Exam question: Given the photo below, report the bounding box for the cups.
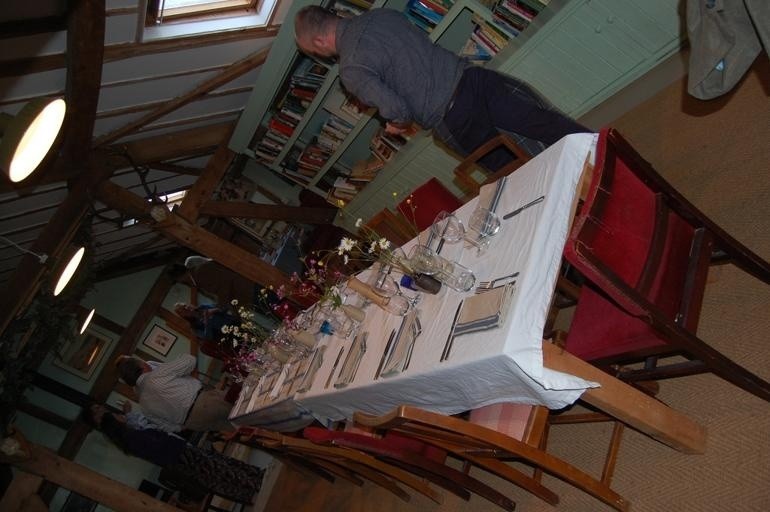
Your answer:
[439,261,480,294]
[467,207,503,236]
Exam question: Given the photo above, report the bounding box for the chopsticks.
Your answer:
[441,297,468,363]
[437,215,451,254]
[374,328,396,379]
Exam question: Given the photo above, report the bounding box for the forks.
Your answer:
[401,315,421,372]
[472,270,521,294]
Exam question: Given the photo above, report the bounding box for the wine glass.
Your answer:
[402,243,460,283]
[227,273,368,402]
[371,271,424,308]
[430,212,492,259]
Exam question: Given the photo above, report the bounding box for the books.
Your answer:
[252,0,551,205]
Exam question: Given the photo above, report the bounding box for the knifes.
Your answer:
[500,195,547,220]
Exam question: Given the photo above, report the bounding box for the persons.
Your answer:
[81,258,277,504]
[294,5,597,175]
